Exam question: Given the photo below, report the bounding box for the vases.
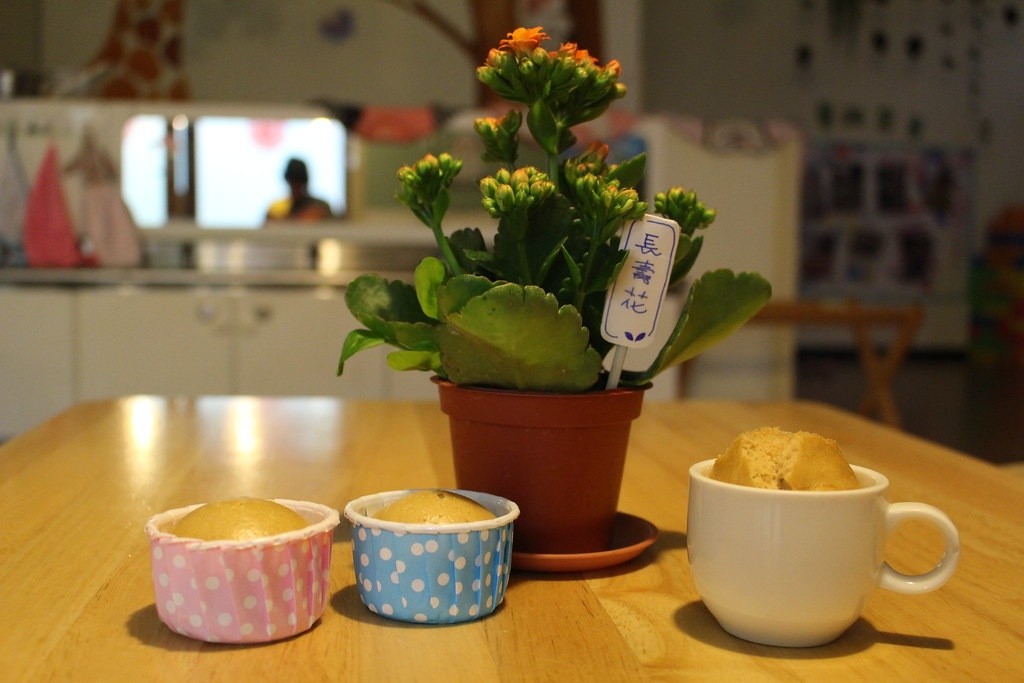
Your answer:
[428,372,662,572]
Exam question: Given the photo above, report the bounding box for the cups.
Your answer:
[684,457,958,649]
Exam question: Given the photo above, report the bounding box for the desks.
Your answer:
[1,390,1024,682]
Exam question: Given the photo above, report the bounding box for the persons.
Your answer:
[263,158,339,227]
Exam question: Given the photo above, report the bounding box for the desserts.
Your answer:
[146,494,340,644]
[343,487,519,623]
[705,426,863,491]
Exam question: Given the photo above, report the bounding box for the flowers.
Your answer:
[331,25,773,396]
